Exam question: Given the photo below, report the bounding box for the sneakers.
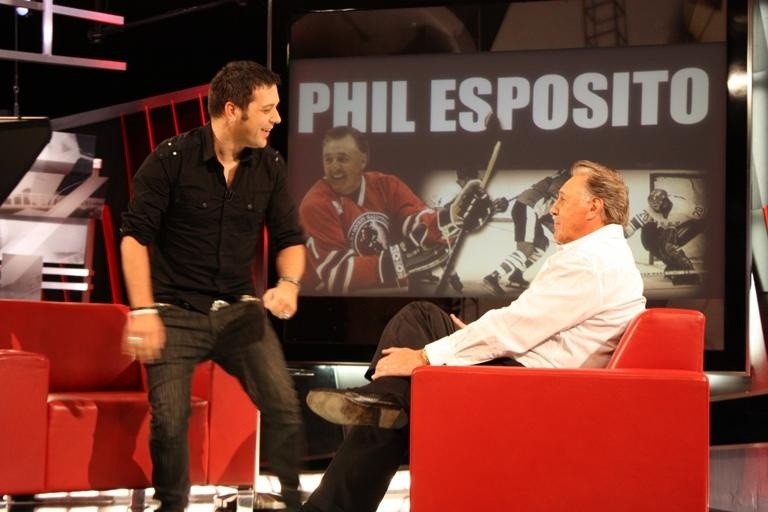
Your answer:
[483,274,505,295]
[509,272,529,287]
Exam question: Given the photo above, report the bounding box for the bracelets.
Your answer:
[420,346,431,365]
[127,304,164,313]
[279,277,301,288]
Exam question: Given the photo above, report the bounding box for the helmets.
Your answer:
[648,188,668,212]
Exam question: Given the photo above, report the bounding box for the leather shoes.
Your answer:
[305,389,409,431]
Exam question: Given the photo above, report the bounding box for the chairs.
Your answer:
[0,297,258,512]
[409,308,710,512]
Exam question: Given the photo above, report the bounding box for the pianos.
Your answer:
[0,127,114,302]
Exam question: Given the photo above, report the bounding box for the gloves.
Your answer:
[378,221,448,283]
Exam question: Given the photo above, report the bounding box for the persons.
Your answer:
[624,188,705,284]
[120,60,307,511]
[253,157,651,511]
[299,125,495,296]
[484,163,574,295]
